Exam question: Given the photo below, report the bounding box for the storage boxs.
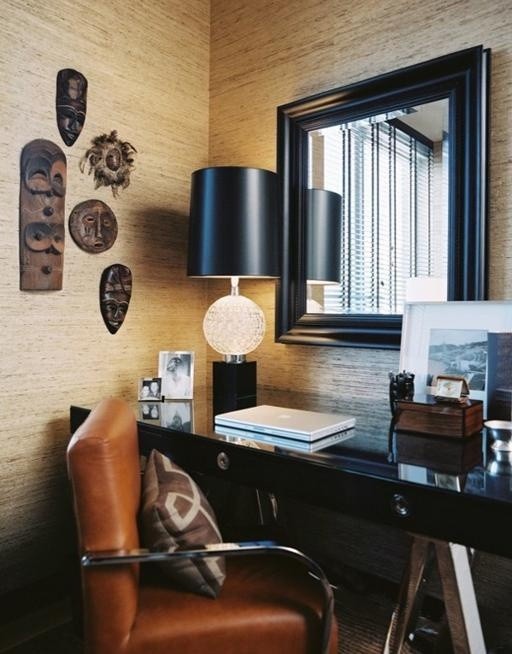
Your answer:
[396,392,484,439]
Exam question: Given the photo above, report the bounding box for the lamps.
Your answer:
[304,188,342,287]
[188,167,283,404]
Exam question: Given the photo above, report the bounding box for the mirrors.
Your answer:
[276,45,489,350]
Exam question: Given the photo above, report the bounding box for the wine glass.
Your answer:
[485,448,512,478]
[483,420,512,452]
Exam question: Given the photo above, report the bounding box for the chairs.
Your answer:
[66,399,338,653]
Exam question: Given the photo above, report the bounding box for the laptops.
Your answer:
[214,403,356,444]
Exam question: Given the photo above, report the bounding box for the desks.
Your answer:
[69,385,512,654]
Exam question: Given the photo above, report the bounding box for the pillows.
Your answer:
[140,449,226,600]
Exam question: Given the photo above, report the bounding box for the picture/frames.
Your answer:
[393,300,512,419]
[138,377,162,403]
[159,353,193,400]
